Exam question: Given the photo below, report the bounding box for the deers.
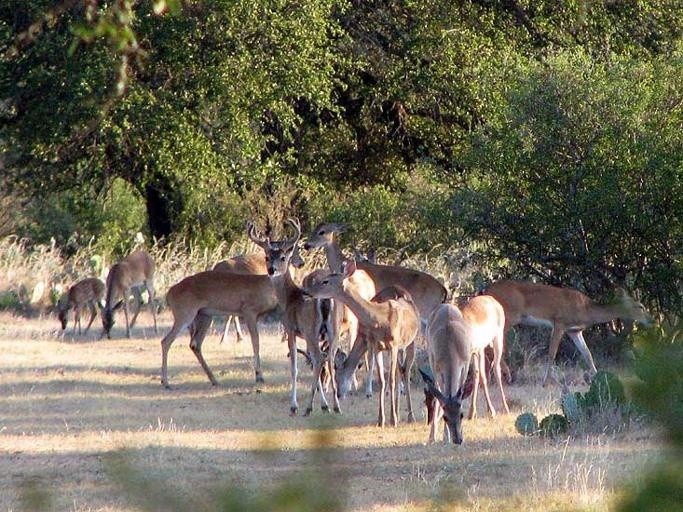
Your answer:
[96,249,159,342]
[159,214,656,447]
[56,277,108,336]
